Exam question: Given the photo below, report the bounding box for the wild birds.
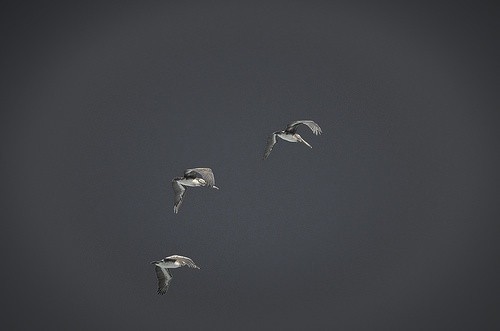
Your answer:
[265,120,323,158]
[172,167,219,214]
[150,254,201,296]
